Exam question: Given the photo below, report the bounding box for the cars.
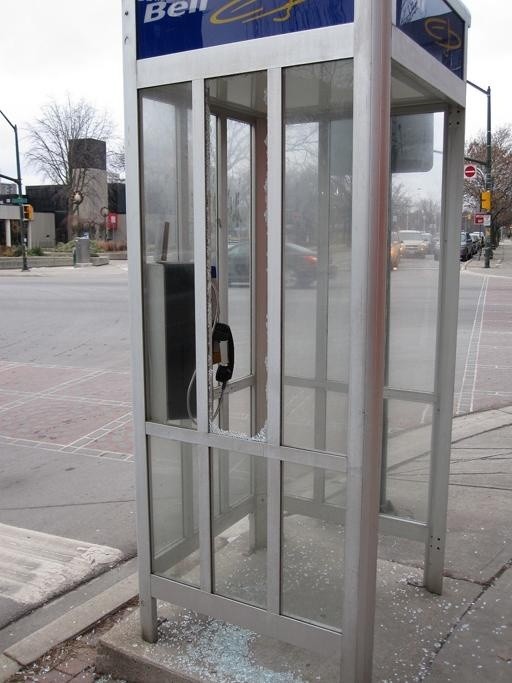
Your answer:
[210,239,335,289]
[390,228,486,272]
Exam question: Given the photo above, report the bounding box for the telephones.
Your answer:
[158,262,235,419]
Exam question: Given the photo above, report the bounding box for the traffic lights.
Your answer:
[480,189,491,212]
[23,205,33,220]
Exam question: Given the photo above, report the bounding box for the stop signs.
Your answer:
[463,164,477,179]
